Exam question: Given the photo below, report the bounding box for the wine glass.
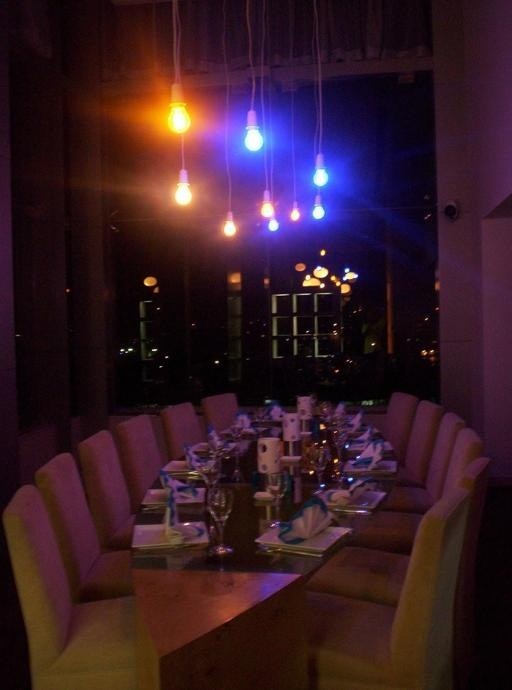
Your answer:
[207,485,235,557]
[268,471,291,530]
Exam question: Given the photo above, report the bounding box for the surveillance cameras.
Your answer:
[444,199,457,219]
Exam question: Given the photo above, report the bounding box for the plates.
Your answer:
[255,522,353,559]
[314,489,386,515]
[142,488,206,506]
[339,423,381,437]
[219,423,269,438]
[160,457,218,475]
[189,438,238,454]
[131,521,210,549]
[344,439,395,454]
[340,457,398,475]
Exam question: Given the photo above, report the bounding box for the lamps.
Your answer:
[166,0,329,238]
